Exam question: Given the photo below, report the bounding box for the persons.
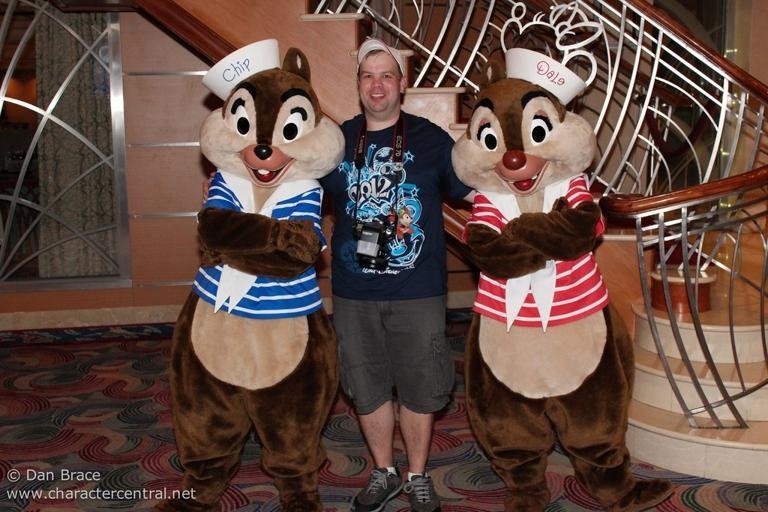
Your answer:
[198,38,480,512]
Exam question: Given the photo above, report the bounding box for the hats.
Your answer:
[358,38,405,75]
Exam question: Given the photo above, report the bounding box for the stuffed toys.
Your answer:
[446,44,677,511]
[167,35,341,512]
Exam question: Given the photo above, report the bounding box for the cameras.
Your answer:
[352,221,393,272]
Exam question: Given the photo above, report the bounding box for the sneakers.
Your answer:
[406,473,440,511]
[351,464,402,511]
